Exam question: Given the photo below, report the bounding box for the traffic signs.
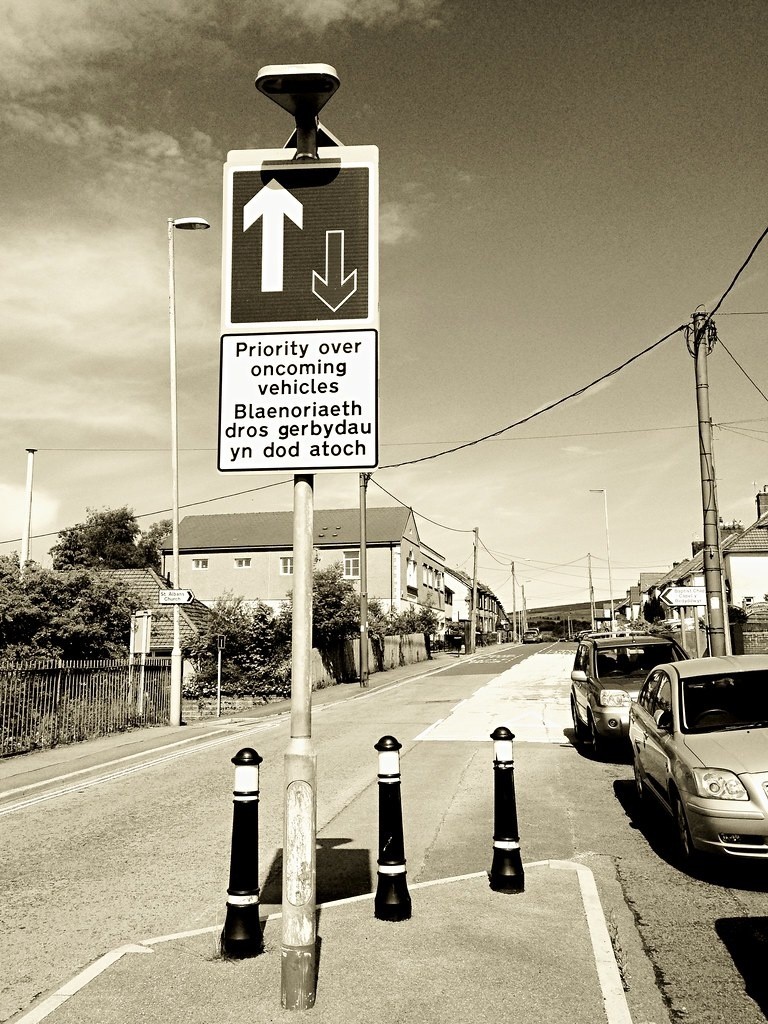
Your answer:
[659,586,708,607]
[158,589,194,604]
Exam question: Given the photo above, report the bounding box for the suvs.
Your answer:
[569,633,690,753]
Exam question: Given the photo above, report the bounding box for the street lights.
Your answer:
[251,61,343,1015]
[167,215,213,728]
[588,488,616,653]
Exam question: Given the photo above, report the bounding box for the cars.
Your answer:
[523,628,543,643]
[629,654,768,878]
[558,629,646,660]
